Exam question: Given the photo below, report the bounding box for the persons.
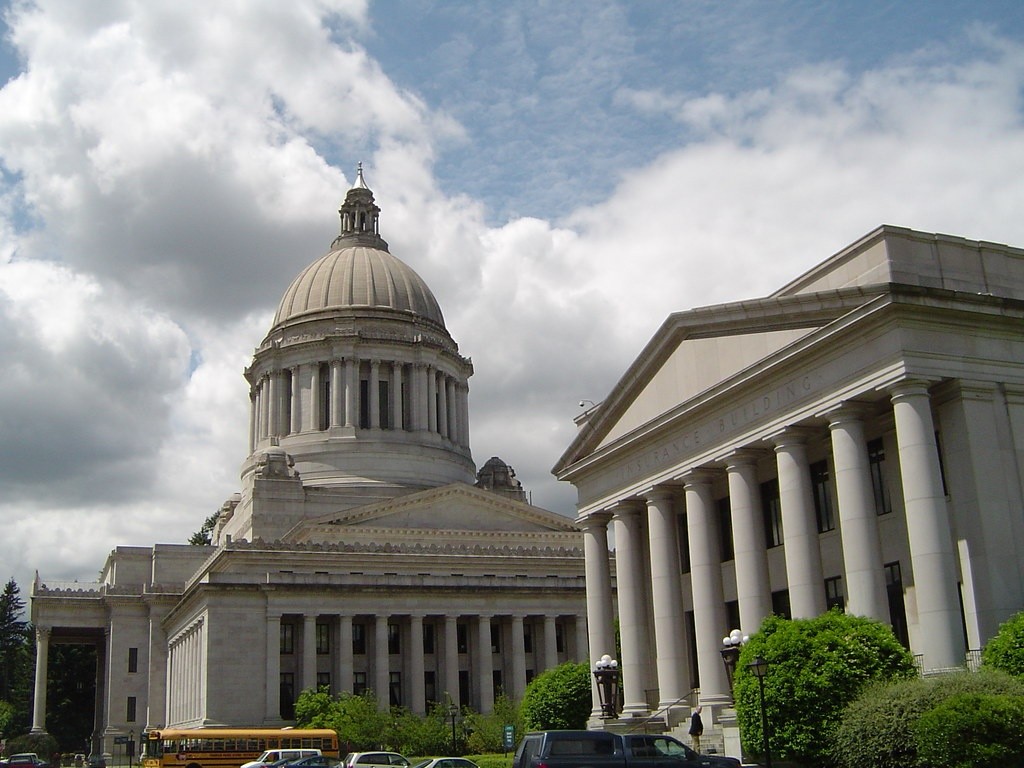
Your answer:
[688,705,703,753]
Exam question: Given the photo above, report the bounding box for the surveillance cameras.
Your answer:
[579,401,584,407]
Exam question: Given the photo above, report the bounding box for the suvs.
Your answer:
[338,751,414,768]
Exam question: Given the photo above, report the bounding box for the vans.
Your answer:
[240,748,322,768]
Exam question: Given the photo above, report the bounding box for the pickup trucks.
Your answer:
[511,728,743,768]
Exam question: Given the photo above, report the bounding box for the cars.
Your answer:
[406,757,481,768]
[0,753,49,768]
[260,755,342,768]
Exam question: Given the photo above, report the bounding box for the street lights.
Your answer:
[448,703,459,757]
[748,654,772,768]
[128,730,134,768]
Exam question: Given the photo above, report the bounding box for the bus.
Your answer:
[142,727,340,768]
